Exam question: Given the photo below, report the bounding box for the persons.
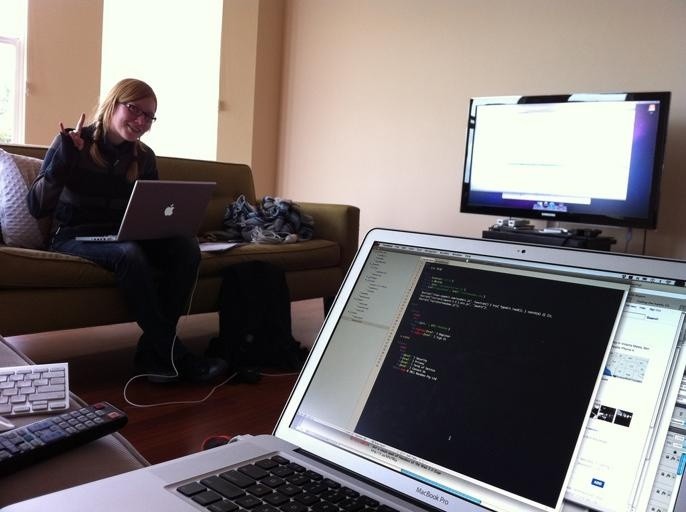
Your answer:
[25,77,218,390]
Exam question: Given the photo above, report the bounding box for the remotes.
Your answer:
[0,398,129,465]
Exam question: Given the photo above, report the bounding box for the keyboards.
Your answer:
[0,361,71,418]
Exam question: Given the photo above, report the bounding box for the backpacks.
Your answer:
[219,259,301,369]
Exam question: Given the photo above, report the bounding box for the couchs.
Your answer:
[0,143,360,367]
[0,333,153,512]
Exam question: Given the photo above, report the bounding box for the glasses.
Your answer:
[124,103,156,123]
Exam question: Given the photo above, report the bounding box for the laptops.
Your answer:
[0,229,686,512]
[73,178,219,243]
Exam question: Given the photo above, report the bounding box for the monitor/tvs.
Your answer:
[458,90,673,233]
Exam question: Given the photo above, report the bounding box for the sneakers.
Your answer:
[134,343,196,383]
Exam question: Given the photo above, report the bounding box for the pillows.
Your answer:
[0,148,50,252]
